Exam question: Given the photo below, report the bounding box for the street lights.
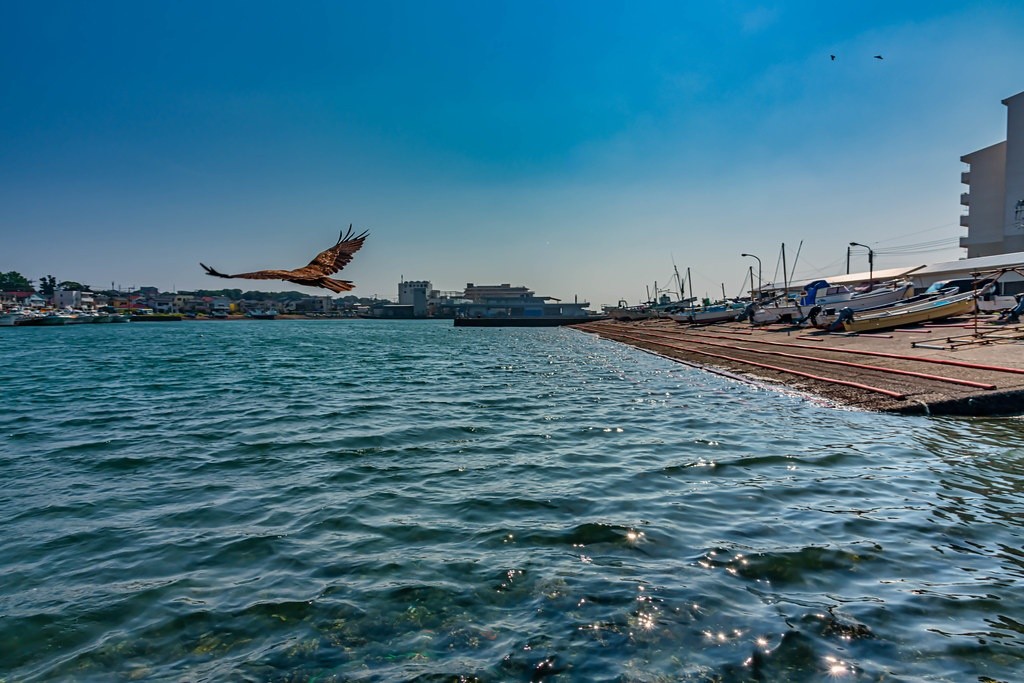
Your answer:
[742,254,761,303]
[850,242,873,284]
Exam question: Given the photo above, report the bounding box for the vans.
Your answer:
[924,278,999,315]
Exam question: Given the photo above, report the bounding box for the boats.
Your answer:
[975,295,1021,314]
[668,304,746,324]
[249,309,278,320]
[816,287,960,331]
[601,295,697,321]
[753,278,915,324]
[15,309,78,325]
[69,309,99,323]
[92,312,117,322]
[0,305,16,326]
[829,289,984,332]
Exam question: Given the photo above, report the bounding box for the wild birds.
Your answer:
[830,55,835,60]
[874,56,883,60]
